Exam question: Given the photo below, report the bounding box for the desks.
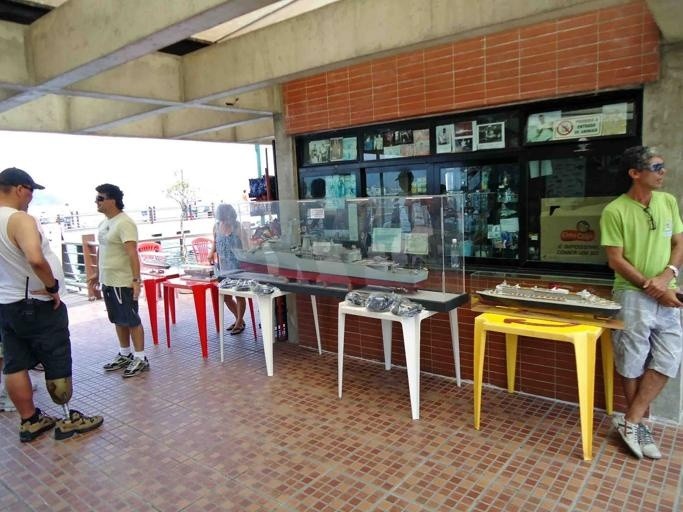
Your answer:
[336,300,461,420]
[472,306,615,461]
[218,284,322,377]
[163,277,219,358]
[143,273,179,348]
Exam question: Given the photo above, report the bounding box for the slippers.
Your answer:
[230,326,244,336]
[226,323,247,330]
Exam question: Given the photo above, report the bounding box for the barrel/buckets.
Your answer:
[444,170,461,192]
[460,238,475,257]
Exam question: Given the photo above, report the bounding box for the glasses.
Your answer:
[635,161,665,172]
[95,196,113,202]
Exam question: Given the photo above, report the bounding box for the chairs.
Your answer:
[137,242,162,298]
[191,237,215,266]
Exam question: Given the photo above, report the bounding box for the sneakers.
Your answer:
[638,423,663,459]
[121,355,151,378]
[32,383,38,391]
[103,352,134,371]
[53,409,106,441]
[611,412,643,458]
[0,390,17,412]
[15,410,62,441]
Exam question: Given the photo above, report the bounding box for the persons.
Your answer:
[207,204,249,335]
[1,167,104,442]
[85,184,149,378]
[390,171,414,228]
[536,114,554,133]
[599,146,683,461]
[311,178,338,230]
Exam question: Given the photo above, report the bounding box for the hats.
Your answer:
[0,167,46,192]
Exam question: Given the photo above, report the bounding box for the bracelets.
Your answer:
[664,264,679,277]
[132,278,141,283]
[45,279,59,293]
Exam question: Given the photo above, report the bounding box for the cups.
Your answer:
[302,235,311,250]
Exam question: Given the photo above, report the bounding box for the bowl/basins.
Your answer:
[309,244,335,256]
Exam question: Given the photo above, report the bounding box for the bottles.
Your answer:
[450,239,459,267]
